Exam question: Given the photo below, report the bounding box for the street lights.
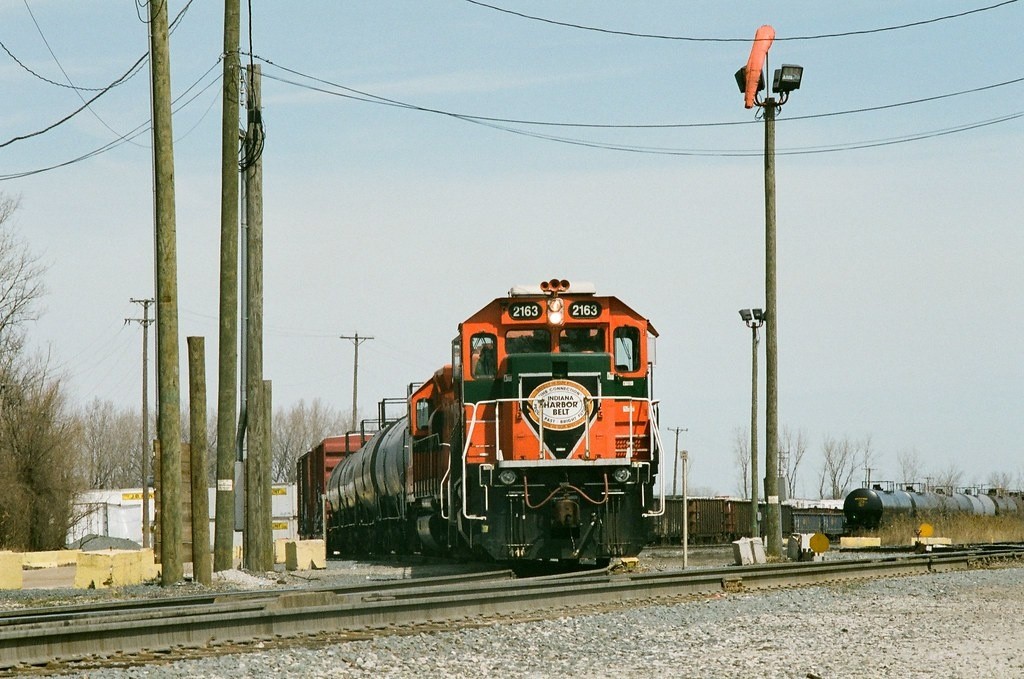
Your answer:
[733,62,805,560]
[737,308,766,536]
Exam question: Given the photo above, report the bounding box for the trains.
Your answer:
[296,275,671,580]
